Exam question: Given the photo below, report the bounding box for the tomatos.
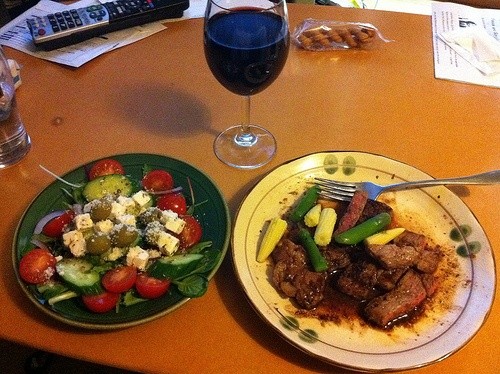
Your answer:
[155,192,186,215]
[142,169,174,196]
[101,266,138,292]
[82,291,121,312]
[41,211,75,236]
[89,158,125,180]
[19,249,57,284]
[135,271,172,298]
[168,215,202,249]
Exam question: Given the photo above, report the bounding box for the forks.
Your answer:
[313,169,500,203]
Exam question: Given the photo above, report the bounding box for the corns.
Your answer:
[304,203,322,227]
[364,227,405,246]
[314,208,337,246]
[256,218,288,262]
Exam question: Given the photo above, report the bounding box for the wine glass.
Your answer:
[203,0,291,170]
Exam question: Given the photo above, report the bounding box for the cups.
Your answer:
[0,48,31,170]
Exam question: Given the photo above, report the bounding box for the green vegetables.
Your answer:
[37,281,79,305]
[169,240,221,298]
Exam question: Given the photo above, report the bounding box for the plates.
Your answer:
[9,151,231,334]
[232,150,497,371]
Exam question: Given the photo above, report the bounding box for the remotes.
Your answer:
[27,0,190,52]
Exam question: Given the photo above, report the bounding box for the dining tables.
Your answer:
[0,3,500,374]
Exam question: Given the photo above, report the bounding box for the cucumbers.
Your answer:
[82,174,132,202]
[147,253,204,279]
[55,258,104,295]
